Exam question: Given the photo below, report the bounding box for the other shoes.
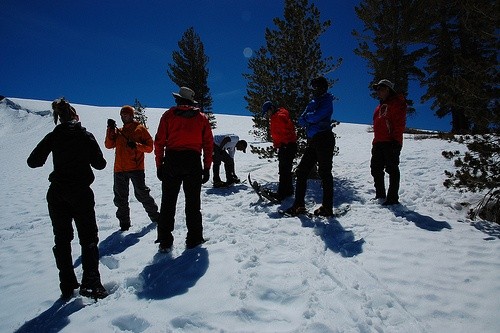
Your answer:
[185,239,205,249]
[59,268,80,299]
[158,241,173,252]
[80,271,109,299]
[227,176,240,184]
[314,206,333,216]
[286,205,306,214]
[213,181,228,187]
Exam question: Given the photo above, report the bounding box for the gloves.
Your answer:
[127,141,136,150]
[201,169,210,184]
[157,168,163,181]
[107,119,116,128]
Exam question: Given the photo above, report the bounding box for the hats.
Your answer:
[241,140,247,153]
[262,101,273,115]
[172,87,198,103]
[120,106,134,117]
[373,79,397,94]
[310,77,328,90]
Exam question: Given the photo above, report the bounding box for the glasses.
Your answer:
[375,86,384,91]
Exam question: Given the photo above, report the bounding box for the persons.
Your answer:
[154,87,214,249]
[212,134,247,188]
[278,75,335,218]
[262,101,297,201]
[27,97,108,301]
[370,79,407,206]
[105,105,160,231]
